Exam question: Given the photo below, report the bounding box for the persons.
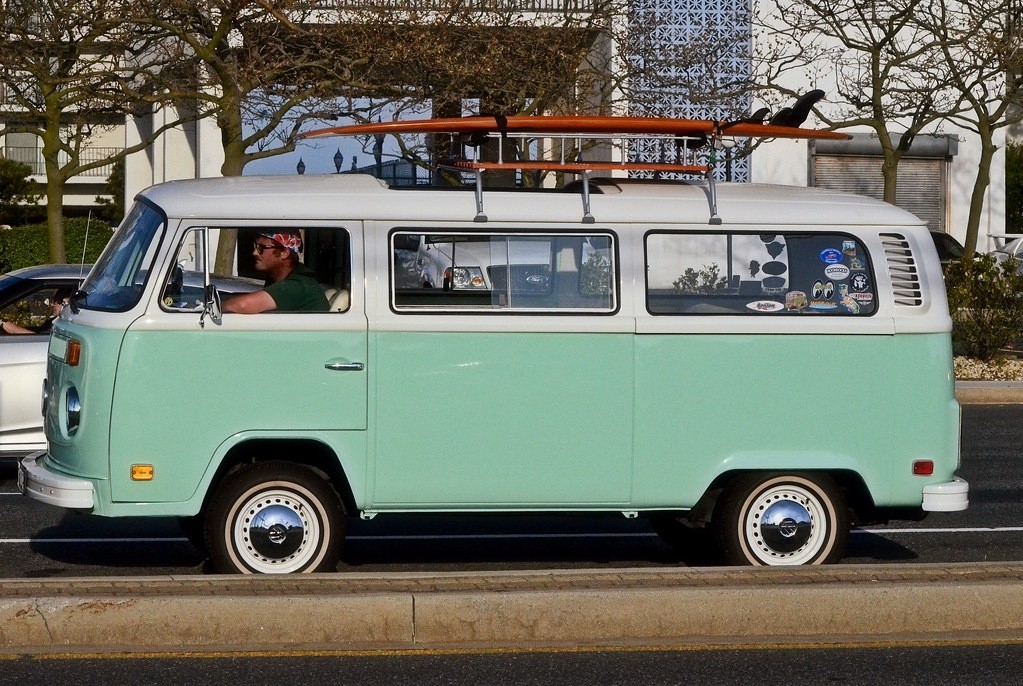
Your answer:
[0,287,76,334]
[221,227,330,314]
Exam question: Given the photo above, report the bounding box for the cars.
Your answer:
[930,229,979,282]
[985,237,1023,278]
[0,265,266,463]
[417,229,610,297]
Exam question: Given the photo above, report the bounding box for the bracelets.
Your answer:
[0,320,8,328]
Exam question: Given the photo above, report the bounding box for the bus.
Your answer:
[29,116,971,577]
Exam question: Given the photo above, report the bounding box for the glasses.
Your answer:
[253,241,284,255]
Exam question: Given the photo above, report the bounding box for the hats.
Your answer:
[259,229,303,257]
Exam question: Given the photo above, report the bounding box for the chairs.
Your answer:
[319,283,350,312]
